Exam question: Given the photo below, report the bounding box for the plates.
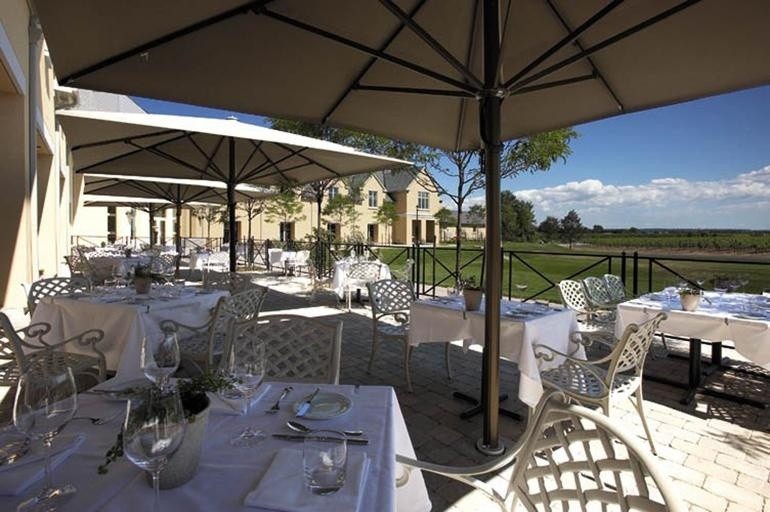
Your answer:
[100,377,153,403]
[292,390,354,421]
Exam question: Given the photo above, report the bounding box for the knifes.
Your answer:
[271,433,370,447]
[295,387,320,419]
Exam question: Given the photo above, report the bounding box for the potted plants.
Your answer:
[446,270,487,310]
[95,377,241,489]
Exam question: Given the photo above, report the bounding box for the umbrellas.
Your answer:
[25,0,770,446]
[81,172,278,273]
[82,194,220,249]
[53,112,416,292]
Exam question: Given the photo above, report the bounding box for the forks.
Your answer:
[68,408,120,425]
[263,386,293,417]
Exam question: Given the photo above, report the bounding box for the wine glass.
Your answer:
[121,388,186,512]
[111,262,126,291]
[10,361,80,512]
[139,331,182,395]
[229,338,269,449]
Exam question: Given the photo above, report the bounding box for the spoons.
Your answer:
[285,419,364,436]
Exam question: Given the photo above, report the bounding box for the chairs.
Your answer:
[393,387,673,510]
[29,275,92,320]
[62,243,418,375]
[555,273,668,368]
[0,309,109,421]
[364,279,453,393]
[213,314,344,383]
[532,308,668,472]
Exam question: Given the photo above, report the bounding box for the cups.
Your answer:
[300,427,348,497]
